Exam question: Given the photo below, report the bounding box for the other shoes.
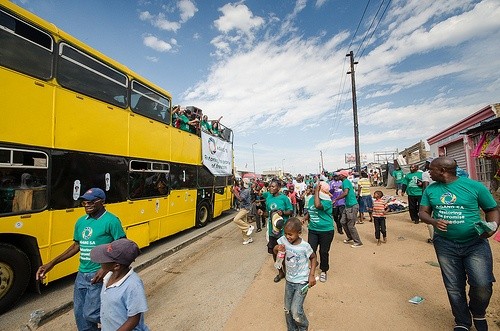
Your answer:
[257,228,261,232]
[351,243,363,248]
[344,239,354,243]
[338,228,343,234]
[454,324,470,331]
[320,272,326,282]
[468,304,488,331]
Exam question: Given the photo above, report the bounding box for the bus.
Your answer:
[0,0,234,315]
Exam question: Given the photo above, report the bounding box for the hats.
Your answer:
[242,178,250,189]
[335,170,349,177]
[317,181,330,200]
[90,238,139,264]
[79,189,106,202]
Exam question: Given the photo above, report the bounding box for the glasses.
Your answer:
[82,199,101,206]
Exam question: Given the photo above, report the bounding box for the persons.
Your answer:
[368,170,378,187]
[454,160,469,178]
[230,174,270,245]
[36,189,127,331]
[283,172,318,218]
[348,172,373,224]
[419,156,500,331]
[392,166,404,195]
[421,160,433,243]
[369,191,390,244]
[12,173,34,212]
[322,169,344,234]
[401,165,424,224]
[258,180,293,284]
[273,218,317,331]
[91,239,150,331]
[335,171,363,248]
[137,94,224,140]
[301,181,334,282]
[150,173,180,194]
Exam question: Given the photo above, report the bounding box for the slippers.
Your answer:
[274,275,284,282]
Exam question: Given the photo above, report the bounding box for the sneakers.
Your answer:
[247,225,254,235]
[243,237,253,245]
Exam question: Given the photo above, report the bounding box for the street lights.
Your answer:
[282,158,286,172]
[252,142,258,174]
